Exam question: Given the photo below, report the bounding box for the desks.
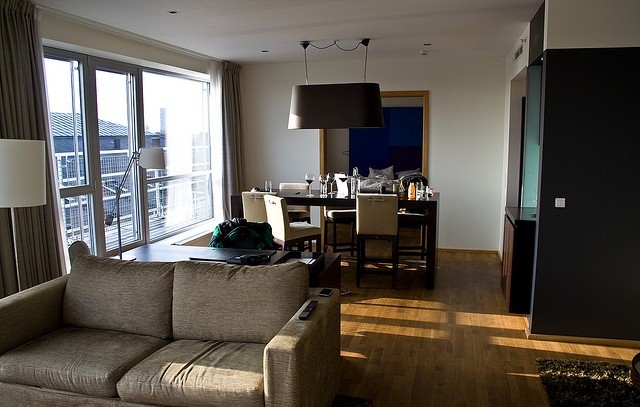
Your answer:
[110,243,342,294]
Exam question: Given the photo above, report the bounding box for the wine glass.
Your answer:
[319,174,327,196]
[327,172,336,195]
[338,172,348,196]
[304,173,316,197]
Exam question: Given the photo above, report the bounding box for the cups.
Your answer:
[264,180,273,192]
[423,186,427,199]
[427,187,434,197]
[416,181,423,201]
[380,182,387,194]
[393,184,400,193]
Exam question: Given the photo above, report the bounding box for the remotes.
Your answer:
[298,298,319,319]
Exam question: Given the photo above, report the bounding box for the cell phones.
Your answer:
[317,285,334,297]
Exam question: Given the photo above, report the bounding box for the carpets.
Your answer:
[534,356,639,406]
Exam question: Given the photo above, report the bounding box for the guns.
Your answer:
[189,254,270,266]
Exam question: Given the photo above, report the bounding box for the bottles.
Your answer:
[349,167,361,197]
[407,182,416,200]
[398,179,405,193]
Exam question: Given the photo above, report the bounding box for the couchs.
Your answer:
[0,253,343,407]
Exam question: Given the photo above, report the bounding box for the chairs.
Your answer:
[241,191,276,223]
[278,182,313,252]
[319,181,356,256]
[354,193,400,290]
[263,193,322,253]
[399,218,425,262]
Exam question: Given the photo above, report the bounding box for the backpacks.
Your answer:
[207,217,274,250]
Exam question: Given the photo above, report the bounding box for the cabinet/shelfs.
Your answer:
[500,213,521,315]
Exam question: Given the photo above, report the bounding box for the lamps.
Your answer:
[1,139,47,292]
[106,147,166,261]
[287,39,386,130]
